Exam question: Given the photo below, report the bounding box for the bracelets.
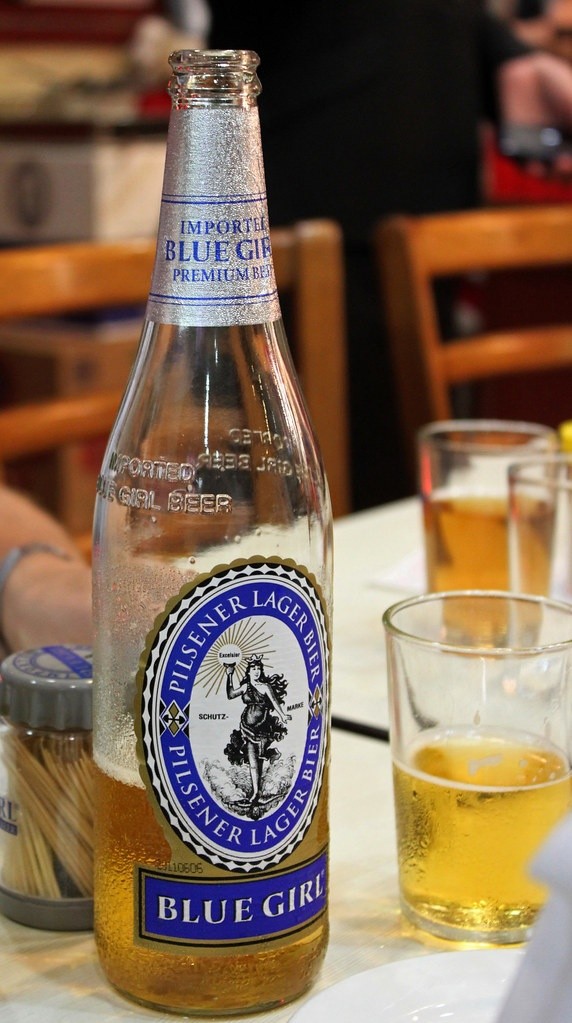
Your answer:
[0,542,68,591]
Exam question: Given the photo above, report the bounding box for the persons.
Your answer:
[0,475,97,654]
[189,0,572,516]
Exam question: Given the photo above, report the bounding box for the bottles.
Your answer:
[90,46,339,1020]
[0,646,93,931]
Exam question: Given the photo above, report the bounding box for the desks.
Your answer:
[0,422,572,1023]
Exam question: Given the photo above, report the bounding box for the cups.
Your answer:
[419,418,559,658]
[507,453,571,700]
[382,590,572,946]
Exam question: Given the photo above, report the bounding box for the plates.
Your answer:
[285,949,527,1023]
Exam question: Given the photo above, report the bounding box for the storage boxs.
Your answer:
[0,114,171,328]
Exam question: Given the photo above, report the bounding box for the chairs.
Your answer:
[0,221,347,576]
[378,207,572,492]
